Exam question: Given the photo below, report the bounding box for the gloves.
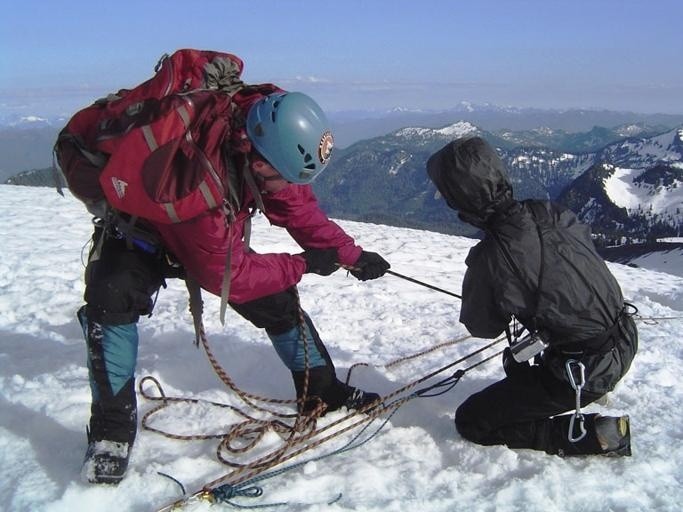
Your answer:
[300,247,339,277]
[350,249,390,282]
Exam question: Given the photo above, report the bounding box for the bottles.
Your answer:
[508,331,549,364]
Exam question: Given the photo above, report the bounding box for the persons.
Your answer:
[421,134,638,459]
[74,76,393,489]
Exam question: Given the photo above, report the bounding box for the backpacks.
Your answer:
[51,47,242,218]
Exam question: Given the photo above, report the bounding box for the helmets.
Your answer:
[246,91,333,186]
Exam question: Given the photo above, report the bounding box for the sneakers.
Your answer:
[83,417,136,484]
[293,371,380,415]
[594,415,631,456]
